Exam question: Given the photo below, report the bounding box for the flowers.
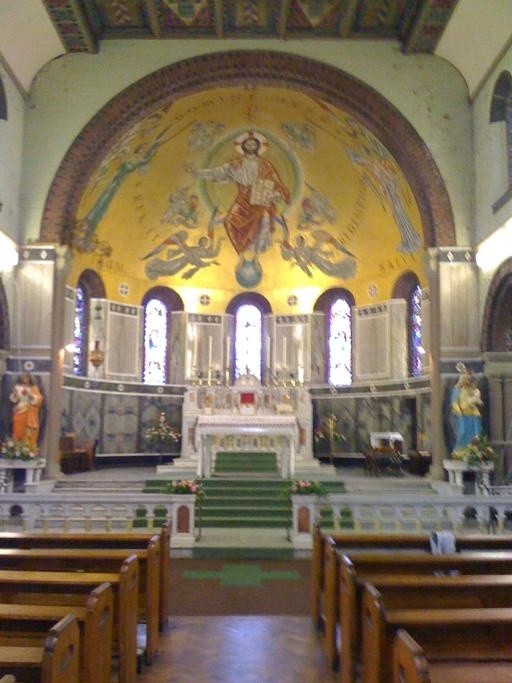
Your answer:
[1,439,38,459]
[168,480,200,493]
[454,434,492,463]
[289,479,319,494]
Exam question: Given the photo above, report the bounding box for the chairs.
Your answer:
[60,433,81,472]
[82,438,98,470]
[363,446,430,476]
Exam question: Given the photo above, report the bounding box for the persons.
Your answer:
[9,371,43,457]
[452,373,484,460]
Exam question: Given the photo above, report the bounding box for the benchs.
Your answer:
[308,524,512,683]
[0,529,171,683]
